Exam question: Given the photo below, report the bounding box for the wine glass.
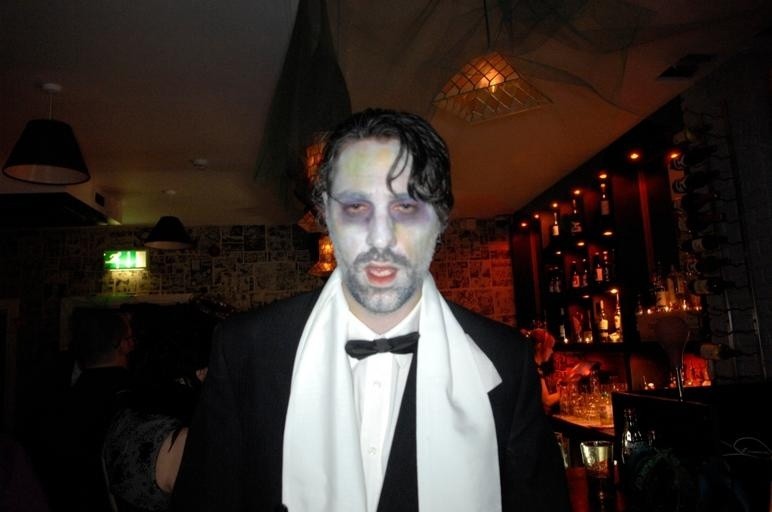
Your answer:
[559,361,628,425]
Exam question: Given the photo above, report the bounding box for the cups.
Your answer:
[579,440,614,490]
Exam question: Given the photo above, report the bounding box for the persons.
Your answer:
[25,309,207,511]
[525,327,563,415]
[170,105,572,511]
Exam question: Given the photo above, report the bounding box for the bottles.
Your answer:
[558,308,567,345]
[569,256,588,289]
[594,249,609,284]
[580,294,595,344]
[548,264,563,294]
[596,299,608,341]
[621,406,656,464]
[598,183,610,222]
[550,211,560,245]
[569,197,582,237]
[613,293,624,336]
[648,259,688,310]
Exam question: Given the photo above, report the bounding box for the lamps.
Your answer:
[141,189,197,250]
[3,83,91,186]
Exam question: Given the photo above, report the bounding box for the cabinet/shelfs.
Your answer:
[523,173,639,352]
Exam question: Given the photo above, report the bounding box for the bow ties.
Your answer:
[344,331,421,360]
